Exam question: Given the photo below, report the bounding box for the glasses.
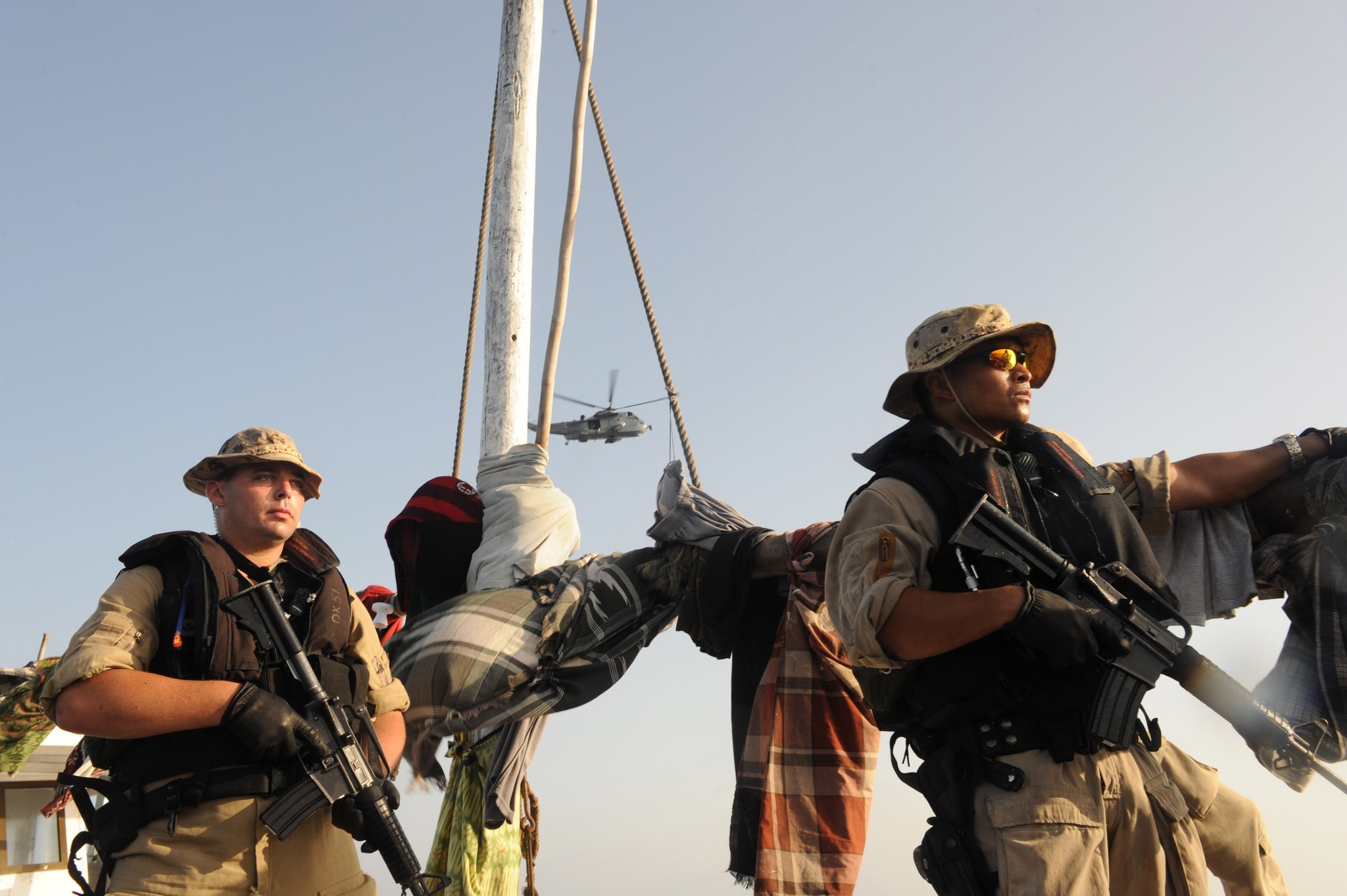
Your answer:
[942,347,1027,371]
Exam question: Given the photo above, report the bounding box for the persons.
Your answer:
[39,427,412,896]
[823,303,1347,895]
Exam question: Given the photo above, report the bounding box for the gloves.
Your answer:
[216,680,330,764]
[331,778,402,854]
[1299,427,1347,461]
[1001,580,1132,672]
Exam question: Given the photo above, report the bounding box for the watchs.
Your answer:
[1273,433,1306,474]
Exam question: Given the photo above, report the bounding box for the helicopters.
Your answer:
[528,367,669,446]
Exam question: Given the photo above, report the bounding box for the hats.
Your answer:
[881,302,1056,419]
[182,426,322,502]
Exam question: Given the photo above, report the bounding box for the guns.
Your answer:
[941,492,1347,805]
[219,582,454,896]
